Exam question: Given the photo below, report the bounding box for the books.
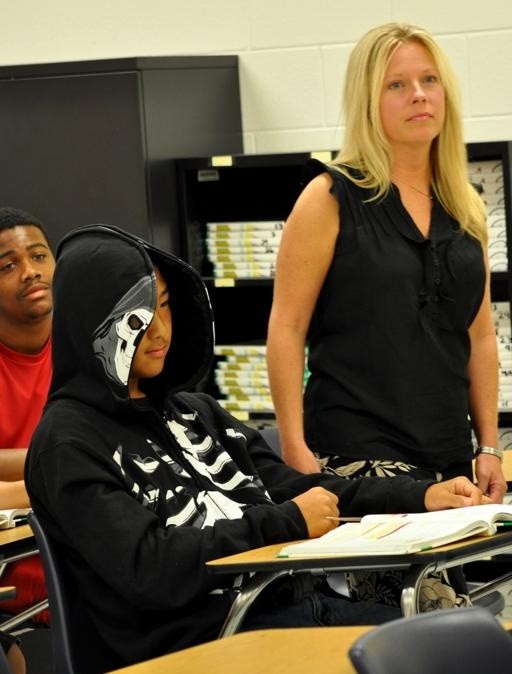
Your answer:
[276,502,512,561]
[205,220,284,280]
[493,301,512,412]
[213,345,313,413]
[0,507,32,529]
[469,161,509,272]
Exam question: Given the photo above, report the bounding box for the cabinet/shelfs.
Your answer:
[175,141,511,451]
[1,55,244,258]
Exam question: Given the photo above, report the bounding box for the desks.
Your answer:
[1,505,511,673]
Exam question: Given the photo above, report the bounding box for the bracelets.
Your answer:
[476,446,504,462]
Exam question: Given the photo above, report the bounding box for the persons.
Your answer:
[265,20,507,614]
[1,632,27,673]
[24,223,494,673]
[0,208,56,624]
